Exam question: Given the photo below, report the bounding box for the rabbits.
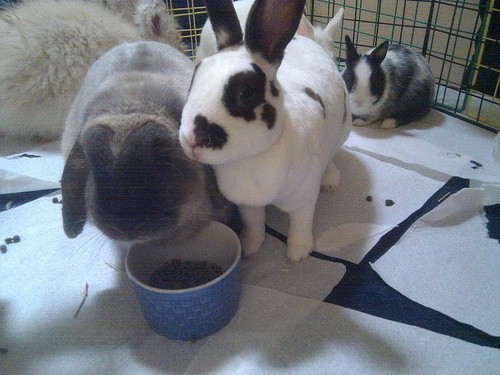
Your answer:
[341,34,436,129]
[0,0,352,265]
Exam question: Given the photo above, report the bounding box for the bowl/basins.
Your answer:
[126,220,242,341]
